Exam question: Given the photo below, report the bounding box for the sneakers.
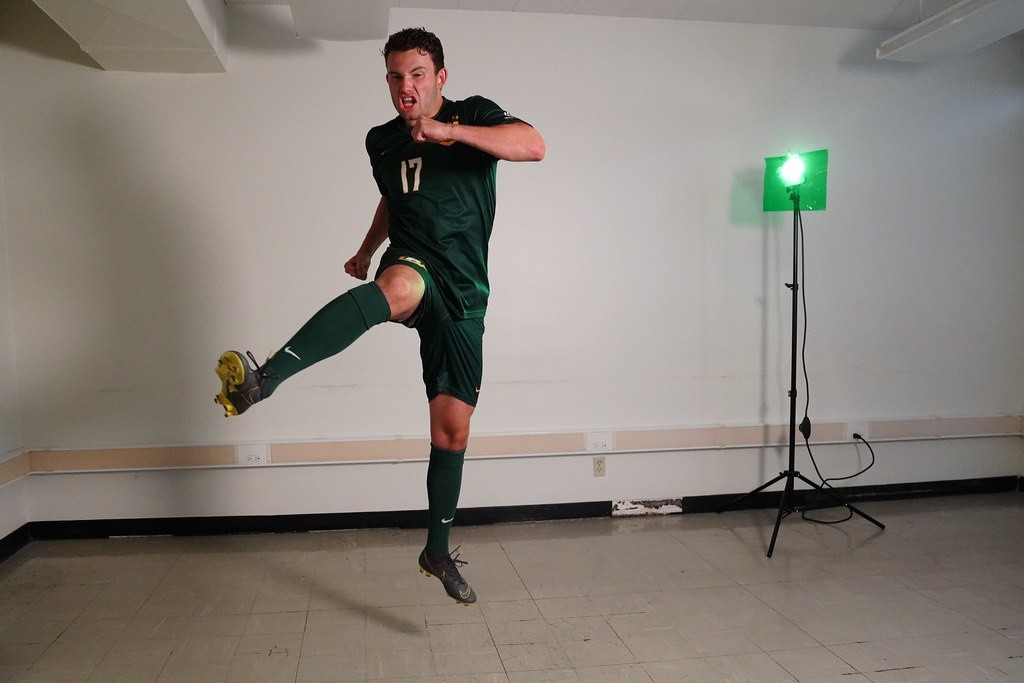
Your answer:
[215,350,277,417]
[418,542,477,607]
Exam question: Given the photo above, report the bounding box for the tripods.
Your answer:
[718,195,886,557]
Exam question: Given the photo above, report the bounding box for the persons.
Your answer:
[215,27,546,607]
